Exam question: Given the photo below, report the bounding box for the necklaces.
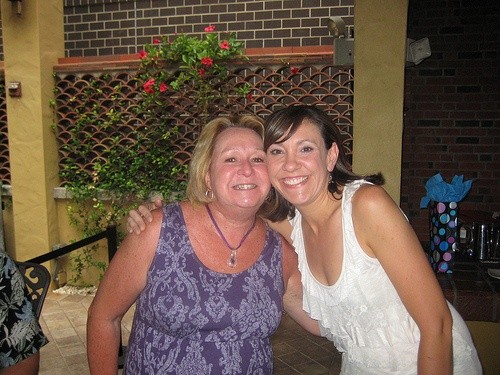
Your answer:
[206,204,257,266]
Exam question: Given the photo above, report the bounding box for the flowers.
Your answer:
[137,25,243,94]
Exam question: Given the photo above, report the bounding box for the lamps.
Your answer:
[326,15,354,67]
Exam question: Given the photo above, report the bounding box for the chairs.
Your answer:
[13,259,53,319]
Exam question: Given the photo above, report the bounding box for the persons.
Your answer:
[126,105,483,375]
[87,115,321,375]
[0,249,50,375]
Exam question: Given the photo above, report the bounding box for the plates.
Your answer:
[488,268,500,280]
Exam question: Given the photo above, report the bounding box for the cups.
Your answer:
[459,218,500,262]
